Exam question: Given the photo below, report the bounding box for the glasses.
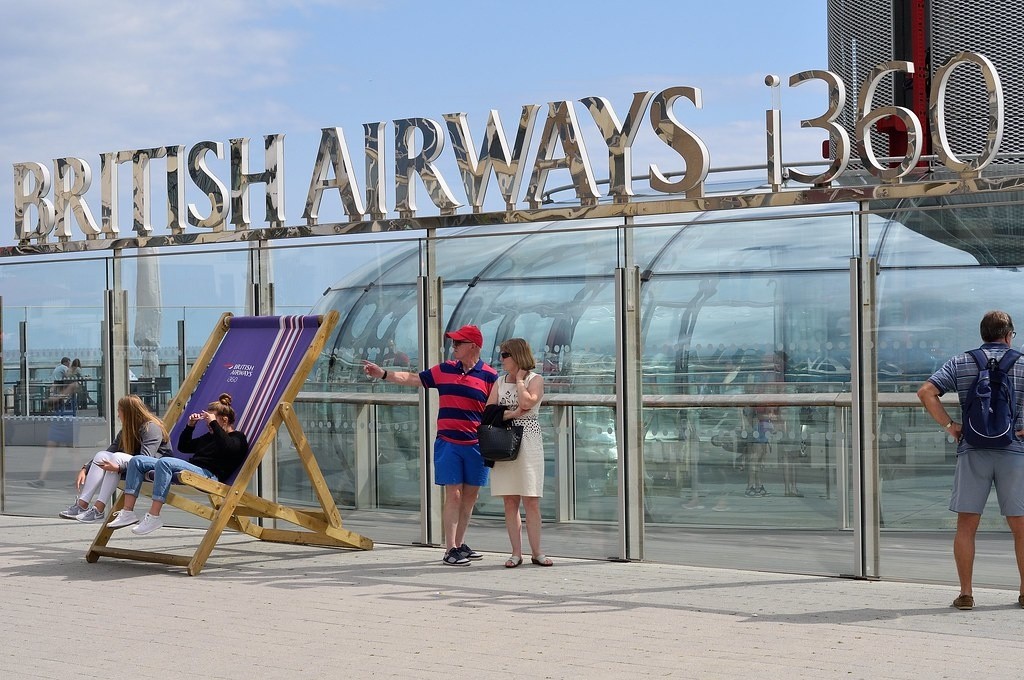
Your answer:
[452,340,473,346]
[1012,331,1016,338]
[499,352,511,359]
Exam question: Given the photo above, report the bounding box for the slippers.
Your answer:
[530,554,553,566]
[505,555,523,568]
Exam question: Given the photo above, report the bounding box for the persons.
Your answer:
[382,339,409,393]
[49,356,95,410]
[652,350,804,509]
[59,394,174,524]
[917,311,1024,610]
[486,338,553,567]
[362,326,498,566]
[107,392,248,535]
[25,379,80,488]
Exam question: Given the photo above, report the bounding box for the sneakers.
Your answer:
[106,509,140,529]
[682,500,705,510]
[26,478,45,488]
[76,505,105,523]
[712,502,728,512]
[132,513,162,535]
[953,594,973,610]
[59,500,90,519]
[1018,595,1024,609]
[744,483,772,498]
[63,480,75,488]
[443,543,484,566]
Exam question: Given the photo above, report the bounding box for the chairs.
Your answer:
[129,375,173,415]
[85,310,374,576]
[15,379,88,415]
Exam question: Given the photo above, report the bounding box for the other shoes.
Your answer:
[89,399,95,404]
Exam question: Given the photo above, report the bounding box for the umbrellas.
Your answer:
[135,247,161,378]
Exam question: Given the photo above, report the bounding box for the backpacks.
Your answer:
[962,349,1024,450]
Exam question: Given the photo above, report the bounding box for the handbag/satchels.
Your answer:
[478,410,524,461]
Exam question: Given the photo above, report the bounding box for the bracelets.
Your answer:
[517,379,524,383]
[118,464,120,475]
[381,370,388,379]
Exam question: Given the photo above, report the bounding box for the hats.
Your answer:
[445,325,483,349]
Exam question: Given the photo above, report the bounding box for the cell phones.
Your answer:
[92,462,108,466]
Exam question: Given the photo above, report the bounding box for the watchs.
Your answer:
[943,420,954,429]
